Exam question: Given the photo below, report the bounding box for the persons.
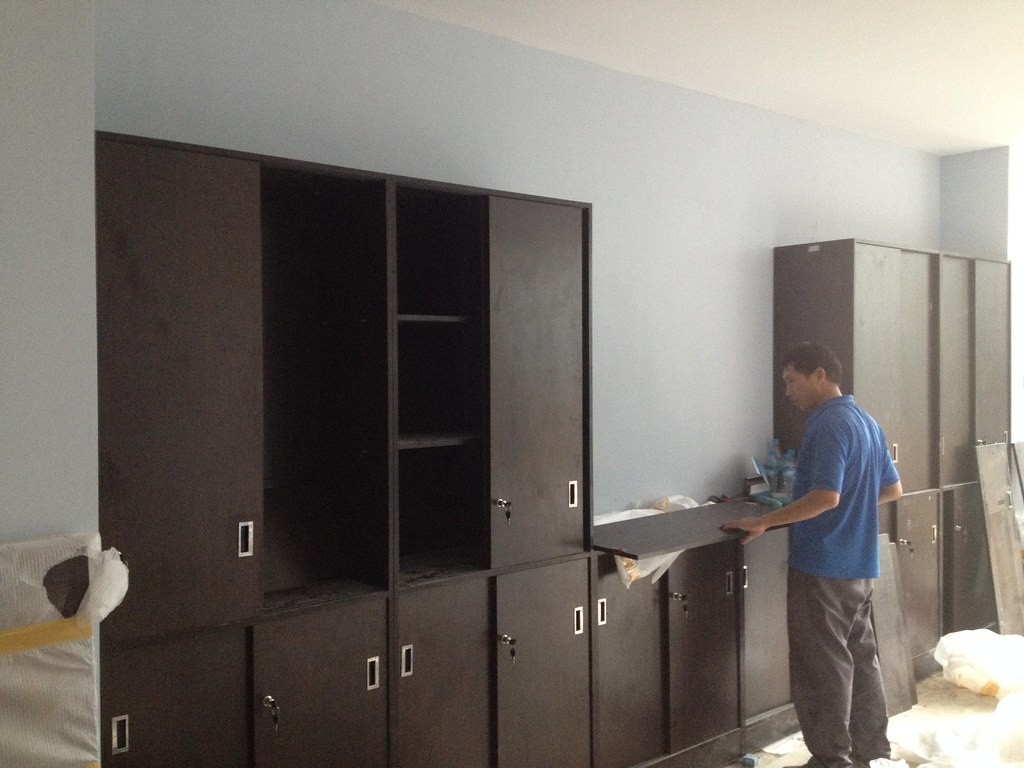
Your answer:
[718,341,904,768]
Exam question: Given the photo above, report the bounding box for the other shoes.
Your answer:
[782,752,871,768]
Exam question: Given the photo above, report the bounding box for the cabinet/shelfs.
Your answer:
[773,239,1011,494]
[391,174,594,582]
[896,492,941,658]
[944,488,994,635]
[664,526,791,755]
[101,553,665,768]
[96,130,393,643]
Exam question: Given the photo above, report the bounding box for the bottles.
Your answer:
[766,439,798,496]
